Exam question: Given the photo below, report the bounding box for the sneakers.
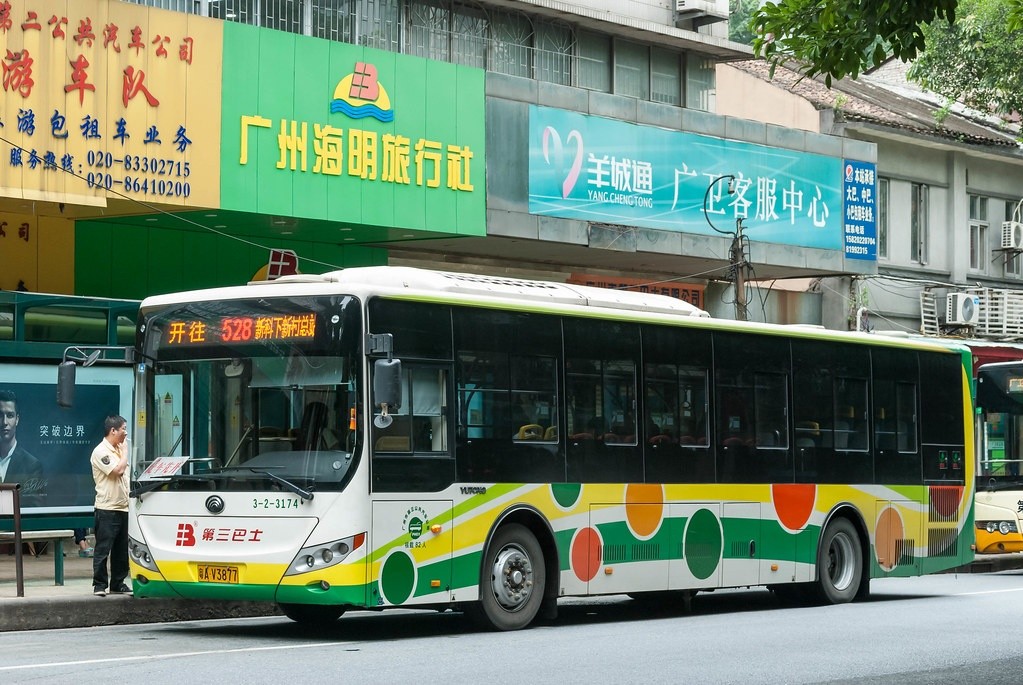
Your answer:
[94,584,134,596]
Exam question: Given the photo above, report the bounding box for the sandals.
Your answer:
[78,547,95,557]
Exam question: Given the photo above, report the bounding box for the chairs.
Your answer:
[372,413,432,453]
[490,393,912,455]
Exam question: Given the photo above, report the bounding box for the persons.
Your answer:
[74,529,95,557]
[292,402,330,450]
[0,390,44,507]
[88,415,134,596]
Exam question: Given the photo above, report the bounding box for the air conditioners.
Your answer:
[1000,221,1022,251]
[675,0,731,20]
[945,292,980,326]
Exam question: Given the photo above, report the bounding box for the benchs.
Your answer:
[0,529,75,586]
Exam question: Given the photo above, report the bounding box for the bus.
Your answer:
[51,260,976,634]
[968,355,1023,571]
